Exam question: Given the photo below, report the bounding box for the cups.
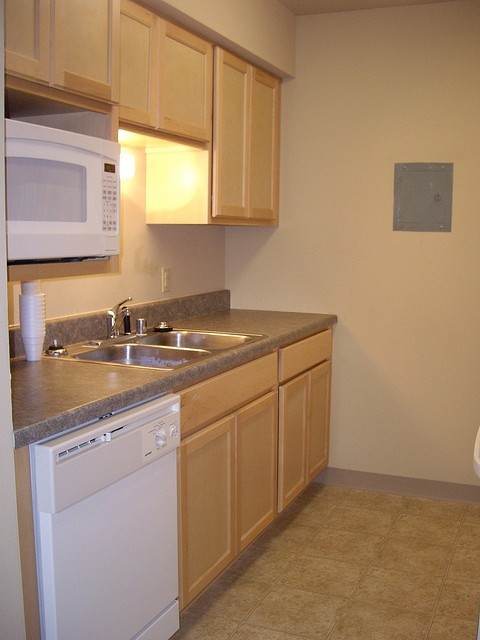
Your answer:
[19,280,45,331]
[19,331,46,362]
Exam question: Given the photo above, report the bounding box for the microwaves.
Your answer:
[5,119,122,260]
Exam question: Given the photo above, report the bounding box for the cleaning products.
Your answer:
[124,308,131,335]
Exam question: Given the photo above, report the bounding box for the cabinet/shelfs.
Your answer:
[179,353,280,605]
[2,0,117,117]
[145,46,280,227]
[118,1,216,148]
[279,328,333,512]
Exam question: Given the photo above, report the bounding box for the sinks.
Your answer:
[70,344,210,370]
[110,332,252,350]
[106,297,133,339]
